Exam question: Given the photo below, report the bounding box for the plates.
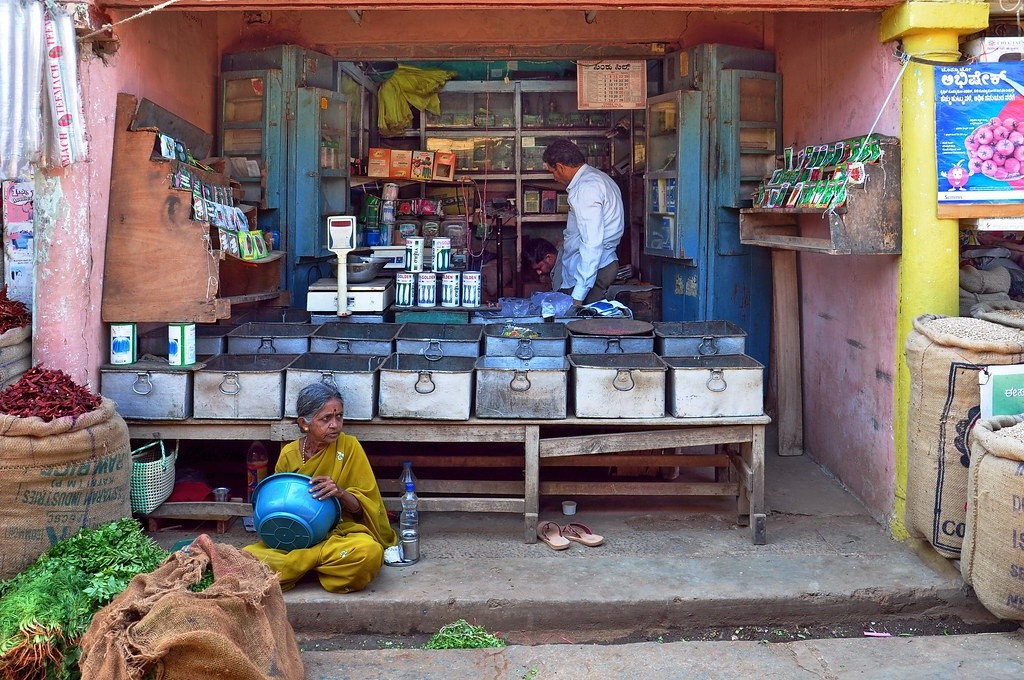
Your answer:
[384,558,419,567]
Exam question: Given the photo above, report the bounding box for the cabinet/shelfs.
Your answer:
[101,92,284,323]
[378,79,632,310]
[220,48,376,310]
[719,68,782,213]
[643,90,704,262]
[740,133,903,255]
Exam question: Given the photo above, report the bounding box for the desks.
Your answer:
[120,414,771,546]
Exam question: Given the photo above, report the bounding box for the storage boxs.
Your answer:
[367,148,455,182]
[426,187,474,216]
[100,312,764,422]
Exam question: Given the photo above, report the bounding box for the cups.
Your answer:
[562,501,577,515]
[212,487,232,503]
[398,528,419,562]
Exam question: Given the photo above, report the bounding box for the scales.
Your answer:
[306,215,396,317]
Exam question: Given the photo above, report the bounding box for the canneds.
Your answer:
[354,182,399,246]
[395,236,482,309]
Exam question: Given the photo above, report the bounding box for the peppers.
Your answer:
[0,362,103,421]
[0,284,32,335]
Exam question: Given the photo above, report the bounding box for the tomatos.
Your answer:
[964,117,1024,180]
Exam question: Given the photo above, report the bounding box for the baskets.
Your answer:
[130,439,180,515]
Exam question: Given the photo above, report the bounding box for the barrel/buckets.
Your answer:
[109,322,139,366]
[168,322,196,367]
[354,182,399,247]
[395,236,482,307]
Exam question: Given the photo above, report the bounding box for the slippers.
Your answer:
[560,523,603,547]
[536,521,570,550]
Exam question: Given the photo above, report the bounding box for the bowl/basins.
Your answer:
[251,472,341,552]
[327,256,391,284]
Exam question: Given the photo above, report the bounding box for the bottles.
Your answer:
[321,140,329,169]
[399,482,419,532]
[329,141,339,169]
[422,215,441,248]
[440,214,467,248]
[399,460,417,517]
[523,92,557,114]
[350,157,367,176]
[394,214,421,246]
[245,440,268,504]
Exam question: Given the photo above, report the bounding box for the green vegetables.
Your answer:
[0,516,213,680]
[421,617,509,649]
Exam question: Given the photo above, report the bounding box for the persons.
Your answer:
[522,237,564,291]
[241,380,397,594]
[542,136,625,305]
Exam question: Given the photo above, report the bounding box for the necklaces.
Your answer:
[302,436,308,464]
